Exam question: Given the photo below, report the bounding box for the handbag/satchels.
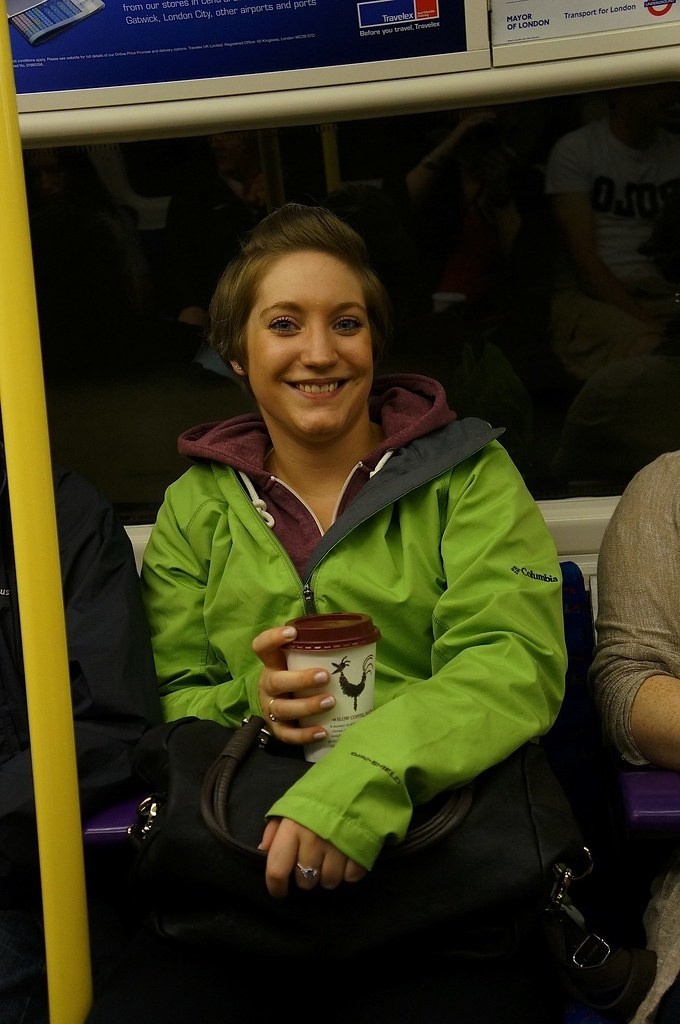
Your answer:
[132,717,657,1018]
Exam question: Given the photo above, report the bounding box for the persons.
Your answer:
[0,435,165,832]
[139,202,570,901]
[587,447,680,1024]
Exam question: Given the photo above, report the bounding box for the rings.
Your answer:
[268,696,281,722]
[296,861,322,881]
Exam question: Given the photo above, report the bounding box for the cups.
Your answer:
[283,616,379,762]
